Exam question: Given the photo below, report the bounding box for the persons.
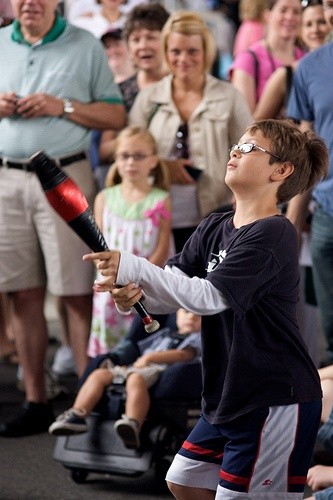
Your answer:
[82,119,324,500]
[0,0,333,500]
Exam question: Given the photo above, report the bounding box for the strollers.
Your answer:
[52,305,202,483]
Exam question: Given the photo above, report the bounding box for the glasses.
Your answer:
[230,142,284,161]
[173,121,189,160]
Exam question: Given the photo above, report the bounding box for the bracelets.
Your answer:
[57,94,75,119]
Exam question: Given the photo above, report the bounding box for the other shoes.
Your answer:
[114,414,139,449]
[4,402,52,438]
[49,409,89,435]
[1,349,20,368]
[17,372,69,402]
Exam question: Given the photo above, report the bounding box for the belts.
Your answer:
[0,151,86,172]
